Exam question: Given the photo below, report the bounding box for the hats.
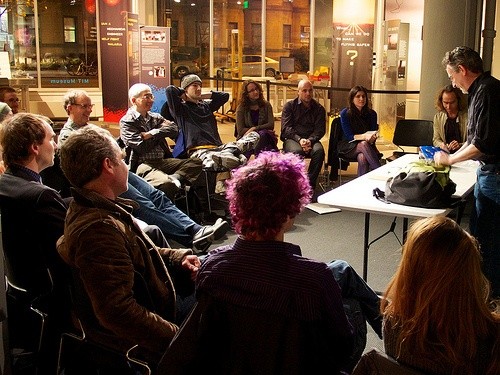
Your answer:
[181,74,202,89]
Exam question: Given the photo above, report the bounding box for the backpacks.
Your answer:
[373,159,462,209]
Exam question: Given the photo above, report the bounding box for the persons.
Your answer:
[192,150,383,375]
[380,216,500,375]
[281,79,327,202]
[0,74,278,375]
[434,46,500,308]
[433,84,468,153]
[336,85,381,178]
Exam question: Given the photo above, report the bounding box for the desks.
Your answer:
[0,75,35,113]
[316,154,484,281]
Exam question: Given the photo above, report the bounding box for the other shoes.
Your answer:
[148,177,182,194]
[212,152,240,169]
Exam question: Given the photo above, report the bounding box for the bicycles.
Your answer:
[65,56,98,79]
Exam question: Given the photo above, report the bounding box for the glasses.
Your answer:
[71,103,95,109]
[140,95,155,99]
[3,99,21,104]
[246,87,258,93]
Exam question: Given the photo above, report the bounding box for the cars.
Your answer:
[213,54,281,80]
[172,56,216,79]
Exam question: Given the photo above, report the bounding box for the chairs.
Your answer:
[328,117,383,187]
[177,168,230,216]
[385,119,433,161]
[3,253,359,375]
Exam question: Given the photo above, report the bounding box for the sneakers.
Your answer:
[192,217,229,254]
